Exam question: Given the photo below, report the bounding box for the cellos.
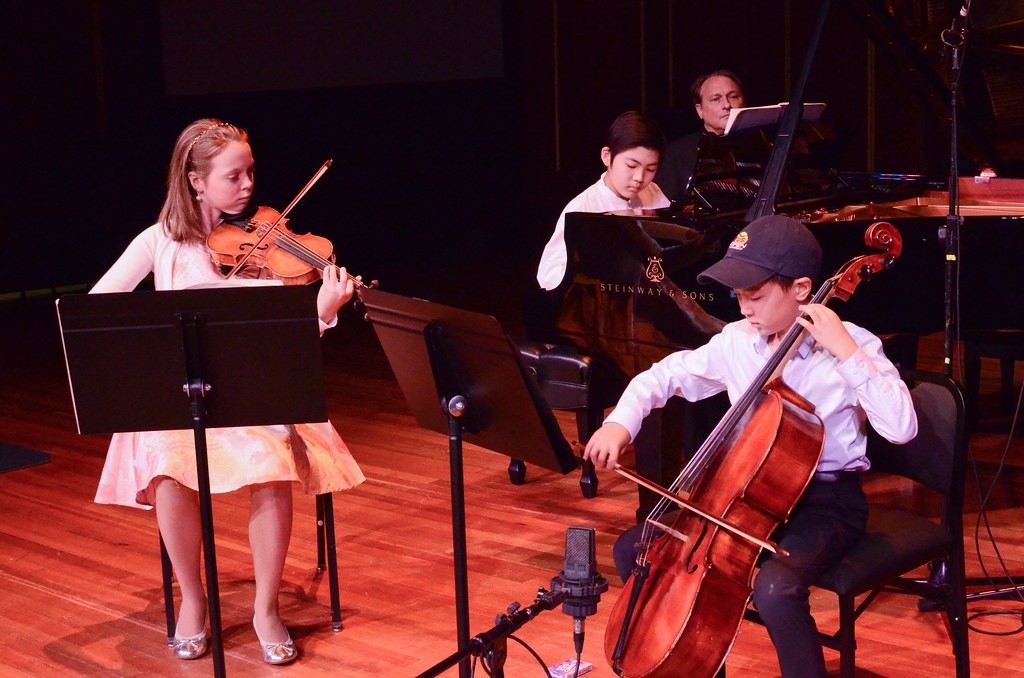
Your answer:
[603,219,902,678]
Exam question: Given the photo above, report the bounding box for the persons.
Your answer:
[583,213,918,678]
[88,119,366,664]
[652,70,778,250]
[536,110,674,291]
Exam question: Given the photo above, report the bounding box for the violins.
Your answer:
[206,206,380,294]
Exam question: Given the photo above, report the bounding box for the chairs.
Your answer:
[746,371,970,678]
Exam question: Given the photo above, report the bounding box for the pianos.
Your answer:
[564,133,1024,592]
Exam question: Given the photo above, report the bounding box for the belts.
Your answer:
[814,472,838,482]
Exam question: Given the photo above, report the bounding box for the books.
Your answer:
[723,102,827,135]
[55,279,284,435]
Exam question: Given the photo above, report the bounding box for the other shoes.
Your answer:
[172,596,208,659]
[253,614,298,664]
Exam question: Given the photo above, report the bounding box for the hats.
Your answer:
[696,216,821,290]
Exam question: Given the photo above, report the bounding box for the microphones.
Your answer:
[562,525,597,654]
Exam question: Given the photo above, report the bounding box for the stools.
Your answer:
[160,490,341,638]
